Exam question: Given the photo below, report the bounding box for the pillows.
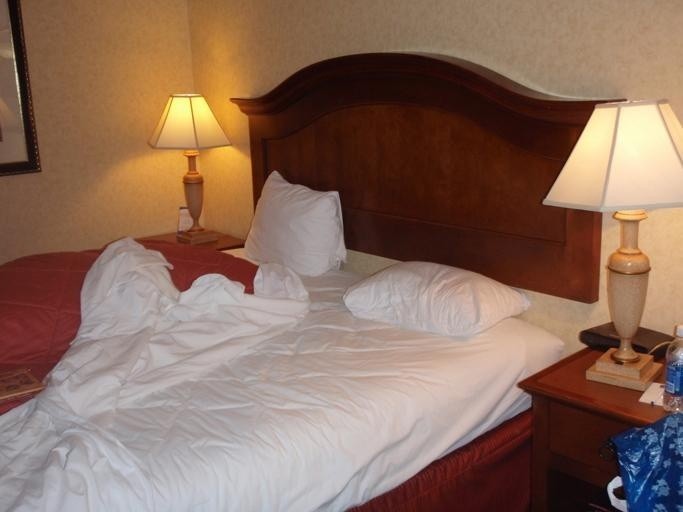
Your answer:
[241,170,344,281]
[342,260,530,338]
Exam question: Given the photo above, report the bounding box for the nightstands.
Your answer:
[141,228,242,255]
[517,345,681,512]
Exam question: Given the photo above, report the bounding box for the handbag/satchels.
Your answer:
[604,413,683,512]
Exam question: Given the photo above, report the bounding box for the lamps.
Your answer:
[147,89,232,245]
[537,97,683,394]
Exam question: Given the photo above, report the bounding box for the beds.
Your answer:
[0,47,601,512]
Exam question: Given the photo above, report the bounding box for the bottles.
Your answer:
[661,324,683,415]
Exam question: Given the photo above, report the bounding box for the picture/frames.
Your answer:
[0,0,44,180]
